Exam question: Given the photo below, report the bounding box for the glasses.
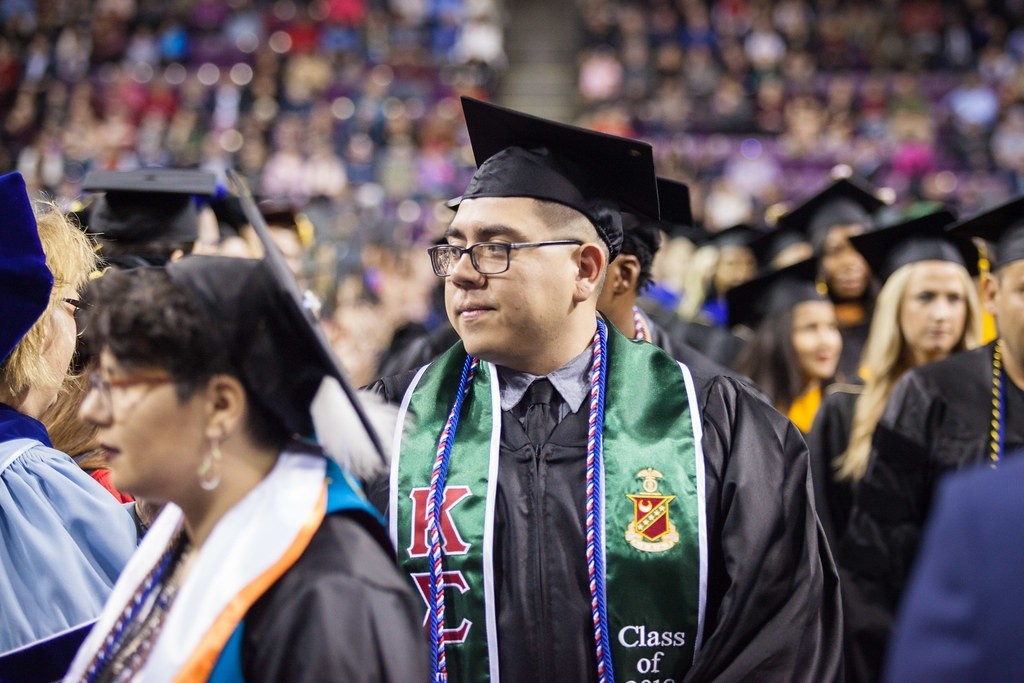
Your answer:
[63,297,97,337]
[427,239,583,277]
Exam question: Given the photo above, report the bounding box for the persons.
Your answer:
[1,0,1024,681]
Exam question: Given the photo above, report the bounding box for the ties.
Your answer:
[521,378,558,457]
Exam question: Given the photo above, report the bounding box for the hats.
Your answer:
[848,210,972,286]
[747,226,809,263]
[226,193,312,247]
[709,223,766,251]
[945,196,1024,346]
[163,169,387,468]
[664,219,712,248]
[725,256,833,328]
[81,167,215,242]
[656,176,694,227]
[776,178,886,234]
[444,95,660,264]
